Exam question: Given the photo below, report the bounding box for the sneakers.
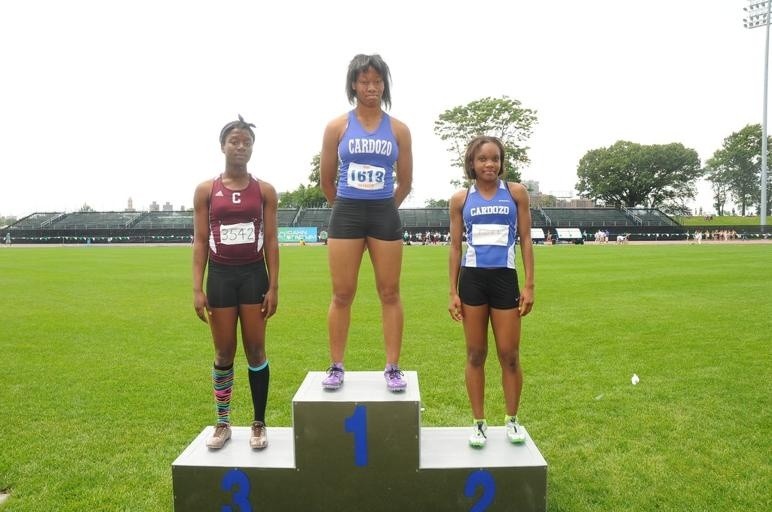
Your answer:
[206,421,231,448]
[321,364,344,390]
[383,366,407,393]
[470,419,488,447]
[250,421,267,449]
[504,415,525,443]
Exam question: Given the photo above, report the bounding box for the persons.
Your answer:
[4,230,12,246]
[449,137,534,449]
[192,114,279,450]
[403,230,450,245]
[594,229,632,245]
[319,53,413,392]
[685,228,737,244]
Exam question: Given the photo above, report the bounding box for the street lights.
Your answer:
[742,0,772,232]
